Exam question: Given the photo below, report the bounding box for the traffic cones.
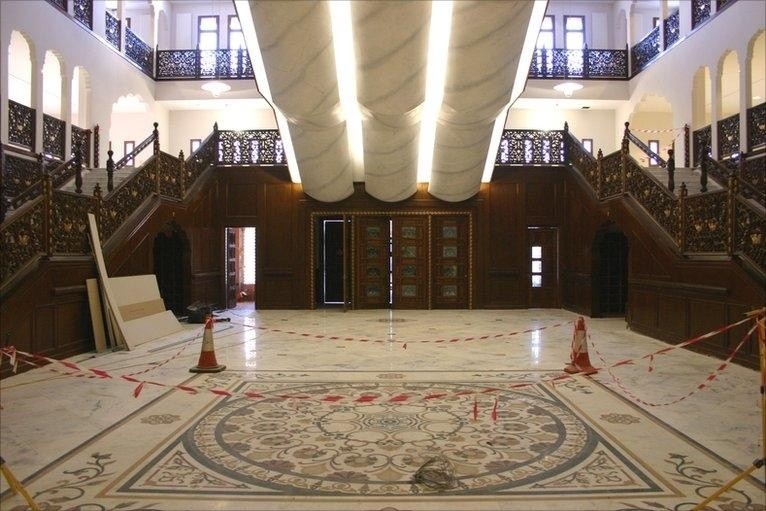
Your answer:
[563,316,597,374]
[188,314,226,373]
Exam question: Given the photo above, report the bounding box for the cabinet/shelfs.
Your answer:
[227,228,244,308]
